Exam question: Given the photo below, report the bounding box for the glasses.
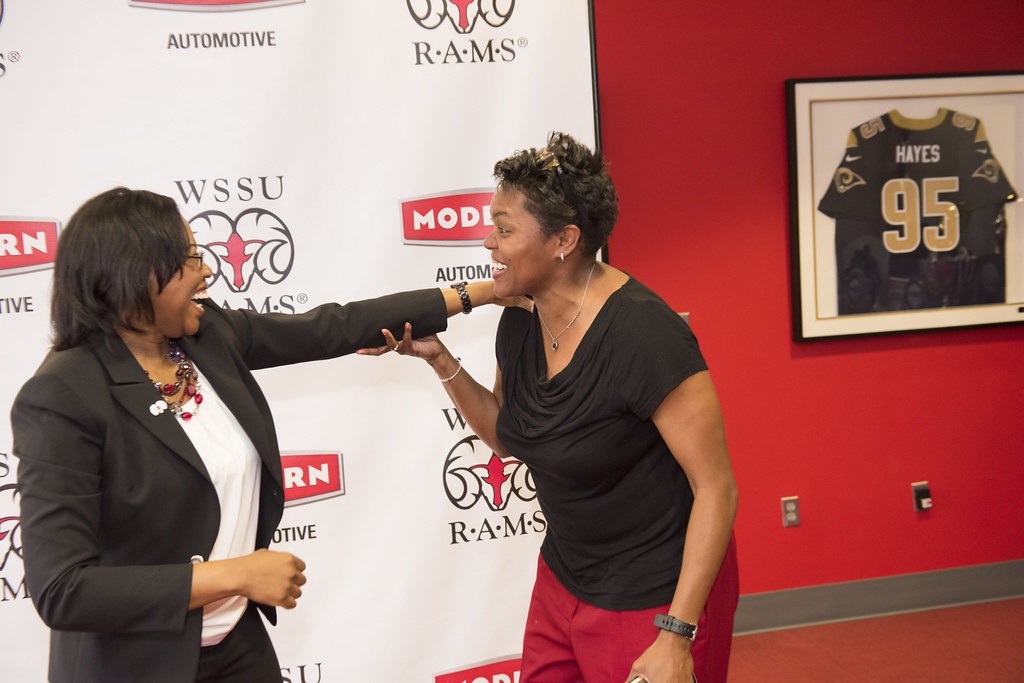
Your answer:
[534,147,575,206]
[176,253,203,269]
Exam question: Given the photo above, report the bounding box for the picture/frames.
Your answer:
[784,70,1024,344]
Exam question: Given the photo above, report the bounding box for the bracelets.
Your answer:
[433,356,462,382]
[654,614,698,641]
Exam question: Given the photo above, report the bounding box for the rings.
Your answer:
[390,344,399,352]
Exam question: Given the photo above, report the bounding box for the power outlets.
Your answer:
[781,496,800,527]
[911,481,932,510]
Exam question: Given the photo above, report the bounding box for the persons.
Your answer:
[354,130,740,683]
[9,187,520,683]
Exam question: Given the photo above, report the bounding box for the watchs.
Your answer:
[450,281,472,314]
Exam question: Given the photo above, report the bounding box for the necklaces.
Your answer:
[537,263,597,351]
[142,337,203,421]
